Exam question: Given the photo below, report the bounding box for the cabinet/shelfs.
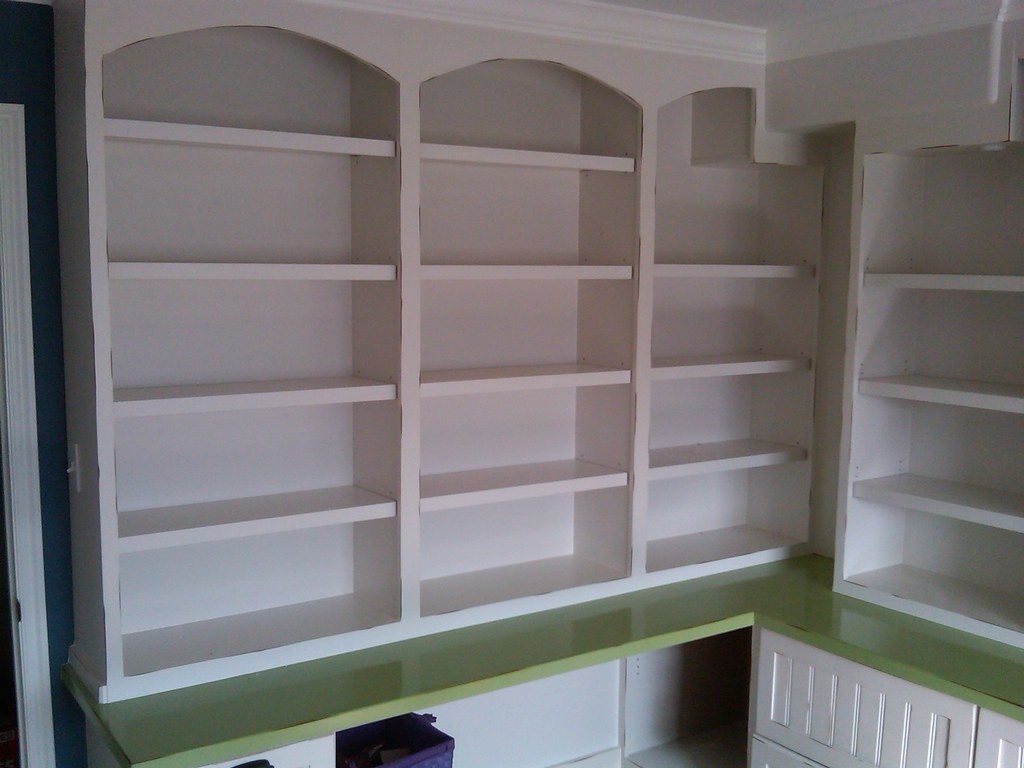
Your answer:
[53,0,834,701]
[829,114,1024,652]
[62,550,1024,768]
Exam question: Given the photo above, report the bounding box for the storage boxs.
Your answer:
[336,712,456,768]
